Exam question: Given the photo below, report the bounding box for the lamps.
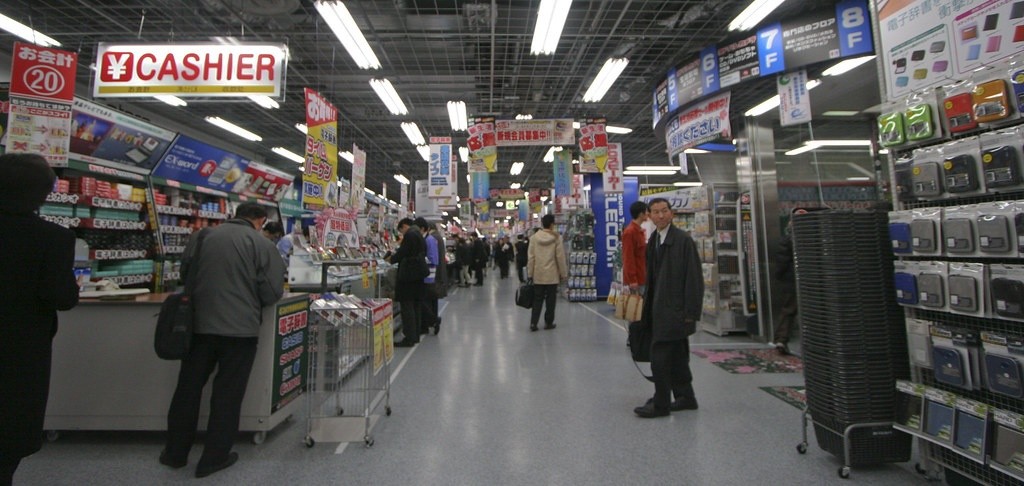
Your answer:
[310,0,383,71]
[531,0,572,56]
[0,11,62,48]
[728,0,787,32]
[368,77,410,117]
[206,115,263,142]
[448,100,469,132]
[401,121,434,163]
[581,58,631,104]
[270,146,307,165]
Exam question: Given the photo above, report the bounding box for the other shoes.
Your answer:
[673,396,698,413]
[545,324,556,330]
[435,316,441,335]
[634,396,670,417]
[420,328,428,333]
[775,340,790,356]
[529,321,539,331]
[395,338,417,348]
[158,448,190,467]
[194,452,239,478]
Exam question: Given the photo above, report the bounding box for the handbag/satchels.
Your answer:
[153,294,193,362]
[615,288,643,320]
[515,278,533,309]
[396,254,431,285]
[628,320,652,362]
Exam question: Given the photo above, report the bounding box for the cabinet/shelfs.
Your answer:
[672,183,765,336]
[149,171,232,293]
[34,158,155,298]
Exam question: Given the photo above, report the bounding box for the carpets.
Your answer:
[763,384,821,413]
[695,347,807,375]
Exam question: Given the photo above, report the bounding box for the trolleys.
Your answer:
[304,297,392,448]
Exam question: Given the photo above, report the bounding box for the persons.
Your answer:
[161,199,284,478]
[385,217,428,347]
[526,213,568,331]
[0,153,79,486]
[774,209,808,354]
[622,201,648,346]
[414,217,442,335]
[260,217,316,283]
[452,227,543,286]
[426,220,445,327]
[633,198,704,418]
